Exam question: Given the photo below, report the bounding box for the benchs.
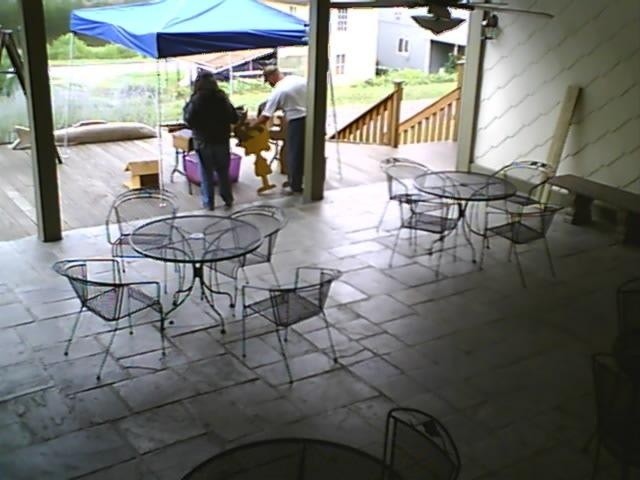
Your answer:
[547,174,640,242]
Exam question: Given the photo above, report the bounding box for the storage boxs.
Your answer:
[122,160,158,190]
[183,152,242,186]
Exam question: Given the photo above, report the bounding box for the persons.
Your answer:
[247,65,308,193]
[182,69,240,211]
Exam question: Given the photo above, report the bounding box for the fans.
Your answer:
[328,0,554,35]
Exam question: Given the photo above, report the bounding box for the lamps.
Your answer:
[480,14,501,40]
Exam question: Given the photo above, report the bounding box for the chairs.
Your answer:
[581,276,640,480]
[106,187,177,295]
[383,408,461,480]
[375,157,576,289]
[242,267,343,385]
[53,258,166,381]
[201,204,288,318]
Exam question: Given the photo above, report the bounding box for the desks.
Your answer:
[170,131,193,195]
[180,438,403,480]
[128,215,264,335]
